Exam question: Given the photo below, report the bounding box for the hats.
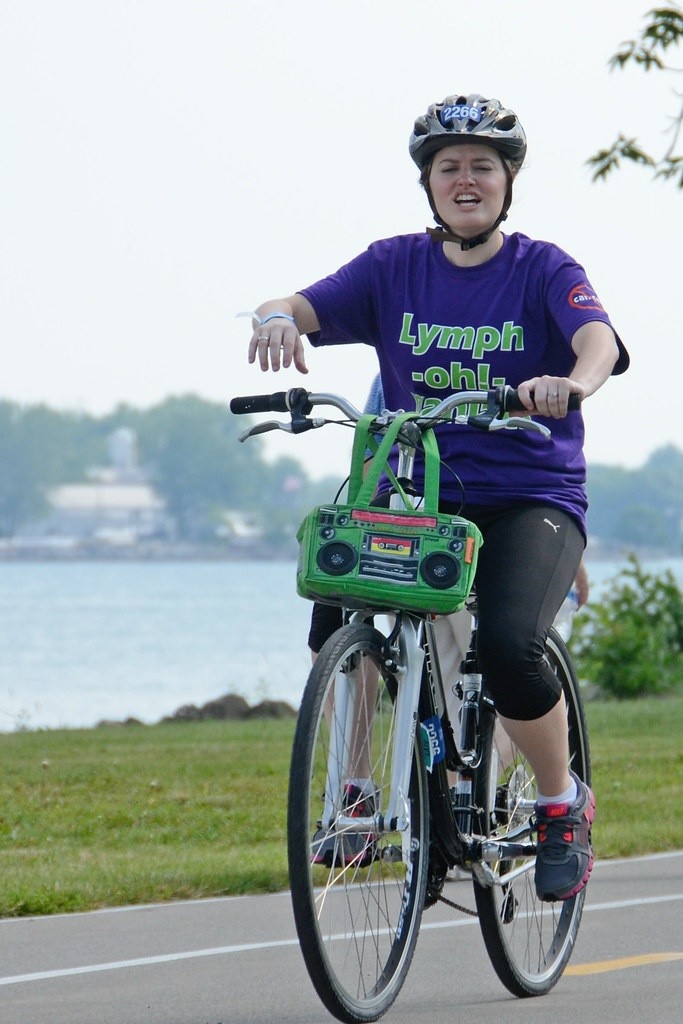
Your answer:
[409,94,527,180]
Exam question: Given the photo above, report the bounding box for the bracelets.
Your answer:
[237,311,295,327]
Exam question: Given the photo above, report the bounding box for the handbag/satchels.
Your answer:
[296,413,484,615]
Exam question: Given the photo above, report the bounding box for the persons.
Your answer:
[236,90,631,902]
[552,558,589,644]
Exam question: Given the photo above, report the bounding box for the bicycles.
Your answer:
[222,384,596,1023]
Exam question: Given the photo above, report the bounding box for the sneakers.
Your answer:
[309,784,380,866]
[528,768,596,903]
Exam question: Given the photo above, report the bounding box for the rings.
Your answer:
[548,392,557,397]
[258,337,269,340]
